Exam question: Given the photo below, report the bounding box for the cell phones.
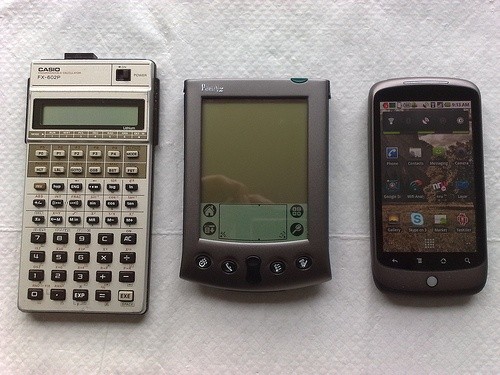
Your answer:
[369,76,488,296]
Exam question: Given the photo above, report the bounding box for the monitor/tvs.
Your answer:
[179,77,333,292]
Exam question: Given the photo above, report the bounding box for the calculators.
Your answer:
[16,51,160,315]
